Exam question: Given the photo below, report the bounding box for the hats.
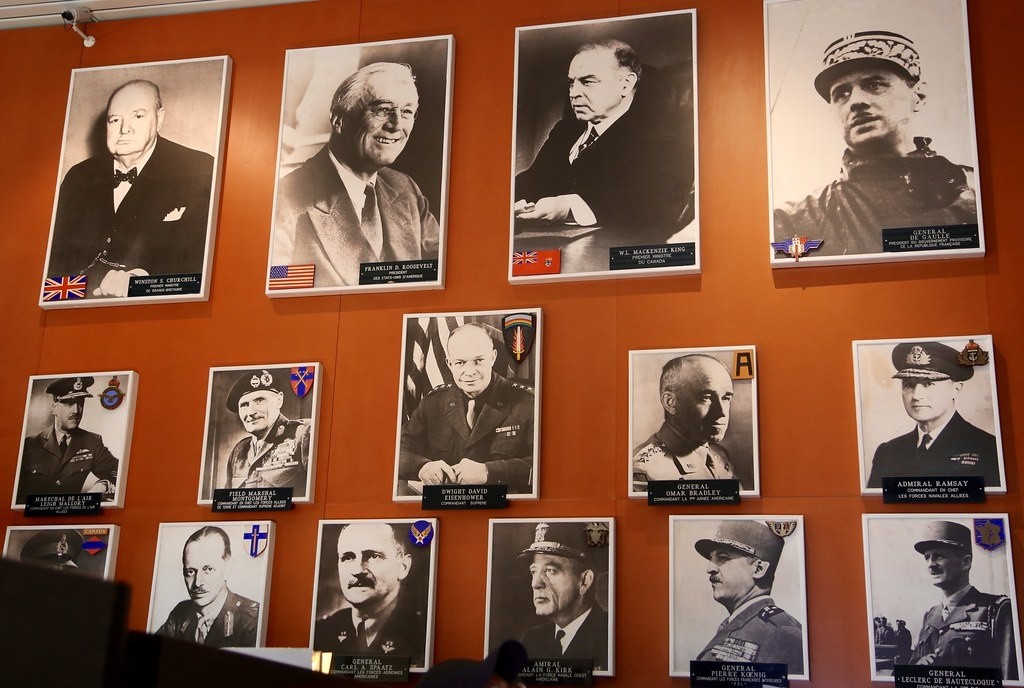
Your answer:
[814,30,921,103]
[891,342,974,382]
[516,523,608,573]
[913,521,972,554]
[694,519,785,570]
[19,529,84,568]
[226,369,280,413]
[45,376,95,400]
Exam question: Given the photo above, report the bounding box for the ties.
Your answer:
[191,617,205,643]
[941,605,950,620]
[914,434,933,461]
[355,622,367,651]
[465,399,477,430]
[361,185,384,259]
[553,630,565,657]
[59,435,67,455]
[716,617,730,632]
[577,126,598,158]
[706,447,719,479]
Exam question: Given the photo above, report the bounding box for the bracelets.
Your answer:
[100,480,108,487]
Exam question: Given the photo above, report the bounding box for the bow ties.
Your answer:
[111,166,138,188]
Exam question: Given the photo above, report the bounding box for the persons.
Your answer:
[420,639,530,687]
[776,30,977,255]
[224,371,310,496]
[632,353,743,493]
[46,78,215,296]
[874,521,1017,680]
[516,40,683,227]
[155,527,260,646]
[516,522,608,658]
[314,525,423,658]
[867,341,1001,488]
[398,323,534,487]
[695,521,802,676]
[52,533,82,569]
[16,376,118,500]
[275,60,439,290]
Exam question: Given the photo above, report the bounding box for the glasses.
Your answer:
[354,103,419,122]
